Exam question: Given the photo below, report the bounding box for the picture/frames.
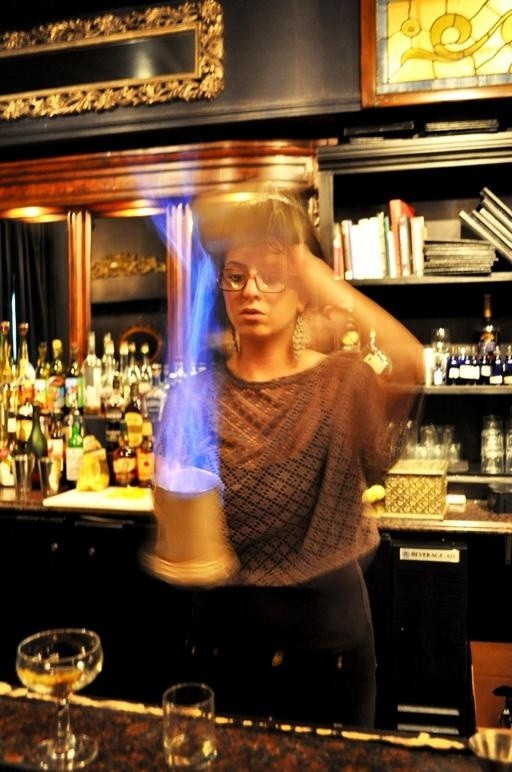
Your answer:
[361,1,512,110]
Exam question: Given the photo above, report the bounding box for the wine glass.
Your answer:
[15,628,103,772]
[432,328,512,368]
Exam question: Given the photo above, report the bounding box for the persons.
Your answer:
[165,182,429,734]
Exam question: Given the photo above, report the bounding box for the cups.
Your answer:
[162,684,219,772]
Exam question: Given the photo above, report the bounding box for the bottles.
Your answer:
[445,318,512,384]
[0,319,162,420]
[505,428,512,475]
[480,414,505,475]
[0,400,158,492]
[402,424,461,464]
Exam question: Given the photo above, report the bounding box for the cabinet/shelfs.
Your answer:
[0,487,154,701]
[316,132,512,734]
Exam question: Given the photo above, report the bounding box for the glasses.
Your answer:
[217,269,286,293]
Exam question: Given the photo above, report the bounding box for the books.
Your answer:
[332,184,512,281]
